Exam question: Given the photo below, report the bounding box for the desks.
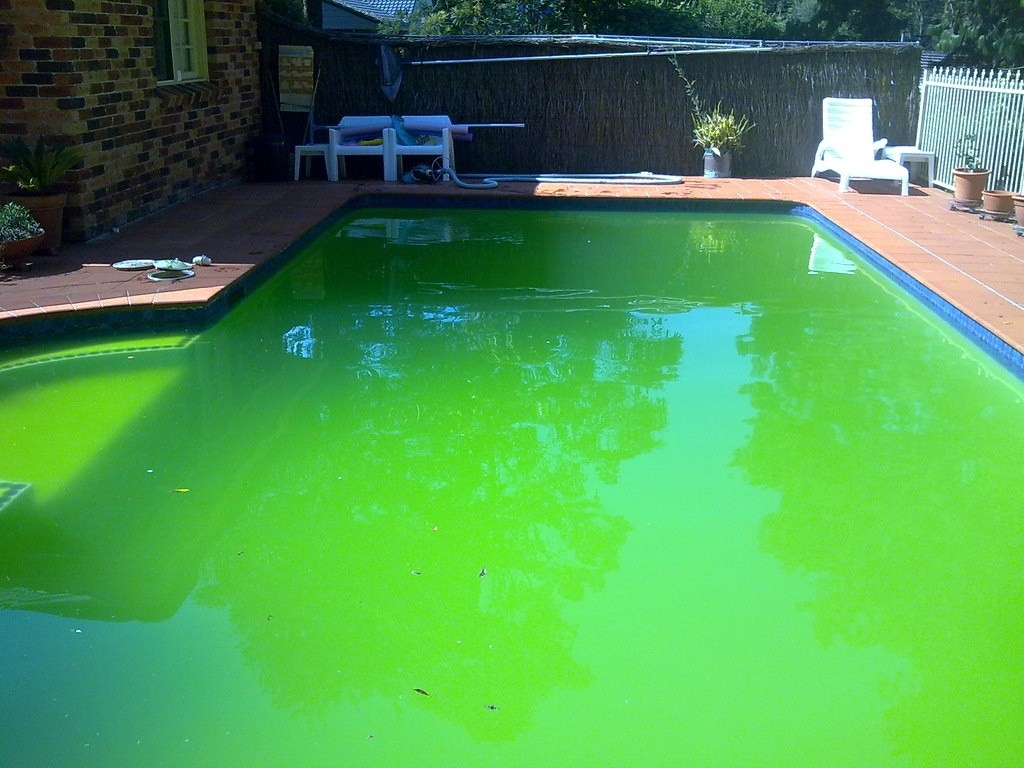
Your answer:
[880,145,935,189]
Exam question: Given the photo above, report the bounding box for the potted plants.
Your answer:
[669,57,759,178]
[952,133,991,202]
[0,133,87,251]
[0,201,45,260]
[981,165,1014,213]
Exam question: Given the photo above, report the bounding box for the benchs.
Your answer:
[811,96,908,197]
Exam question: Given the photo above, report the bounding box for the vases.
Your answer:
[1011,195,1024,228]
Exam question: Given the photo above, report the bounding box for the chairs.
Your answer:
[336,218,451,246]
[808,234,855,274]
[293,110,456,182]
